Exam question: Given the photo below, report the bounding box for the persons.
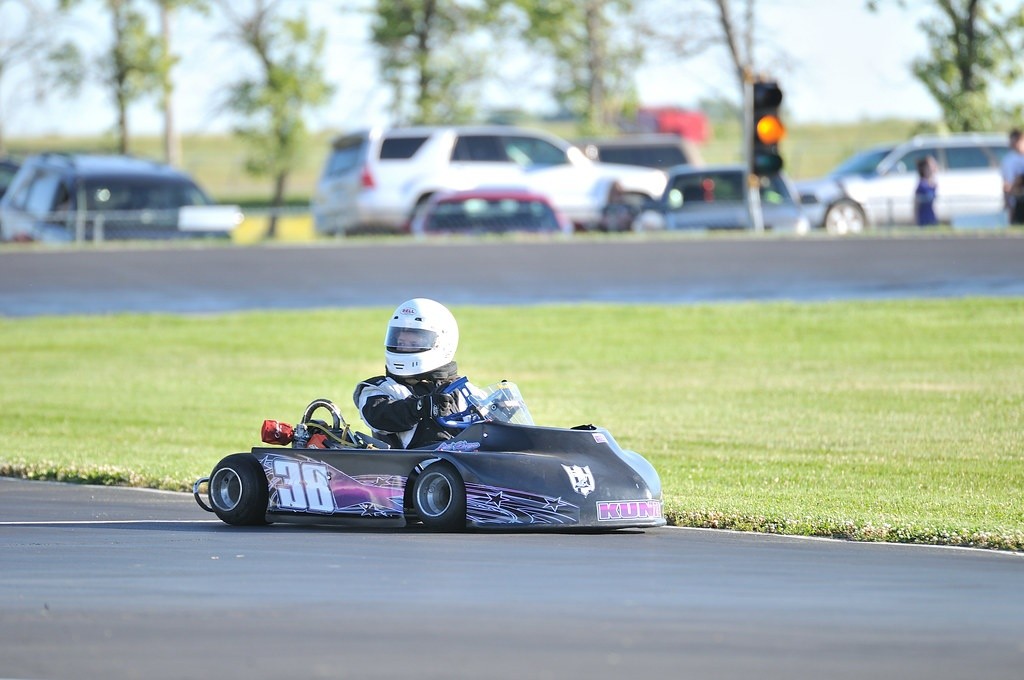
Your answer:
[912,156,940,225]
[353,297,510,452]
[997,128,1024,225]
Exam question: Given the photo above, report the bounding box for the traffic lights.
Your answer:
[751,81,787,177]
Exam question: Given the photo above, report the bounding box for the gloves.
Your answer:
[484,388,520,423]
[416,382,454,418]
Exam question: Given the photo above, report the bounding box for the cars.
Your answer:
[802,130,1011,233]
[654,163,811,234]
[573,130,706,171]
[315,121,669,235]
[410,187,575,235]
[0,148,236,246]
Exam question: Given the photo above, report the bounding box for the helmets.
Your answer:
[385,298,459,376]
